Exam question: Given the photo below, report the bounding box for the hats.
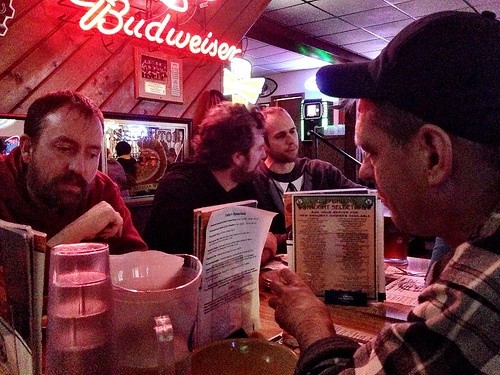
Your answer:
[315,10,499,148]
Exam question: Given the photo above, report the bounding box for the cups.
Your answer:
[46,242,113,375]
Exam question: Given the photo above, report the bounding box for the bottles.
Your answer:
[287,229,293,271]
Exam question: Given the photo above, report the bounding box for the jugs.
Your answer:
[114,255,203,375]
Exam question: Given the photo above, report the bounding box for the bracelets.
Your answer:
[263,247,276,259]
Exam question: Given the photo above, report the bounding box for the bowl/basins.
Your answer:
[192,338,298,375]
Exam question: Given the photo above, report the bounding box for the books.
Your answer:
[0,221,46,375]
[194,189,385,345]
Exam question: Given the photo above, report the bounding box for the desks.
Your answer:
[258,252,431,356]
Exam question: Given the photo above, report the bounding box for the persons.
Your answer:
[106,141,175,196]
[0,91,148,255]
[147,101,371,274]
[266,11,500,375]
